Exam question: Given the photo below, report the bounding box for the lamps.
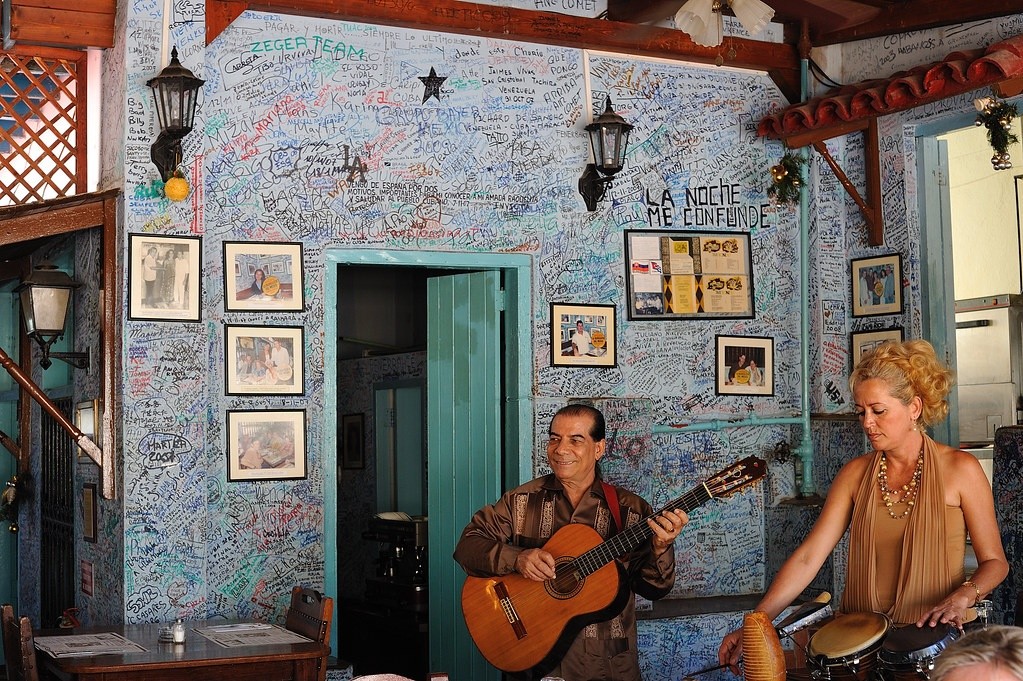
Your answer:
[577,95,635,211]
[674,0,774,67]
[12,247,92,375]
[145,45,206,181]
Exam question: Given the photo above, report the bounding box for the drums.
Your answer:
[804,611,896,681]
[877,621,961,681]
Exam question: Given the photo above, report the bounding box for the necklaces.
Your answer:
[877,452,923,518]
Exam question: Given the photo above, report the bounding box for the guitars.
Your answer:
[461,454,768,681]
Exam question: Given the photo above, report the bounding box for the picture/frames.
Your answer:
[623,228,757,319]
[715,334,776,398]
[850,327,905,373]
[343,411,367,469]
[549,302,617,367]
[225,408,308,479]
[851,253,905,316]
[224,324,306,396]
[128,232,204,322]
[222,239,305,313]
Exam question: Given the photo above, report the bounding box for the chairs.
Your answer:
[0,603,39,681]
[284,586,333,681]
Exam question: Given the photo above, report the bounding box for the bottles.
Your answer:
[172,618,185,643]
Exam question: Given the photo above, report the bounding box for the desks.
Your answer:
[33,618,331,681]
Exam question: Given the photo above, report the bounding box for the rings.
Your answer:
[667,528,675,533]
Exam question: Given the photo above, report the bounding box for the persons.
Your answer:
[141,247,190,311]
[239,430,294,469]
[728,352,763,386]
[572,320,593,356]
[859,265,895,306]
[453,404,689,681]
[252,270,273,302]
[238,340,290,385]
[718,339,1010,676]
[635,294,663,315]
[929,623,1023,681]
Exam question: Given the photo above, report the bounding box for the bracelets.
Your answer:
[961,581,980,604]
